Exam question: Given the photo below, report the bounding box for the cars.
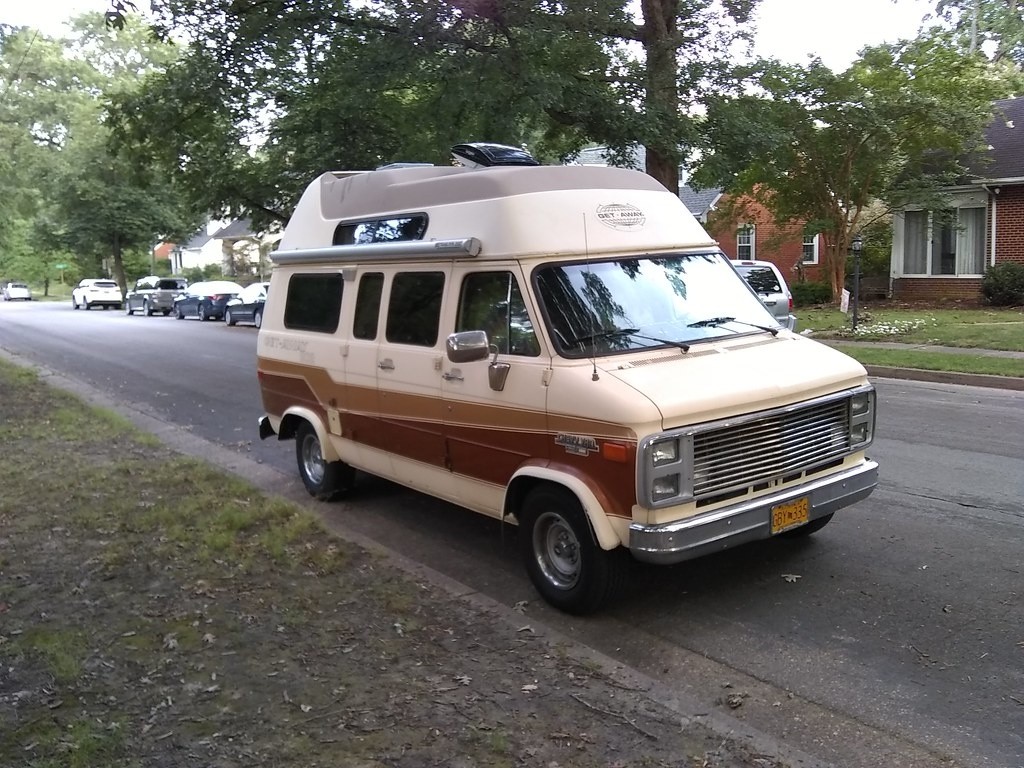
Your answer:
[725,259,797,336]
[227,281,272,328]
[73,278,124,310]
[4,283,33,301]
[124,274,190,319]
[171,281,246,322]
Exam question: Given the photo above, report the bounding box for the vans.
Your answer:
[255,142,880,617]
